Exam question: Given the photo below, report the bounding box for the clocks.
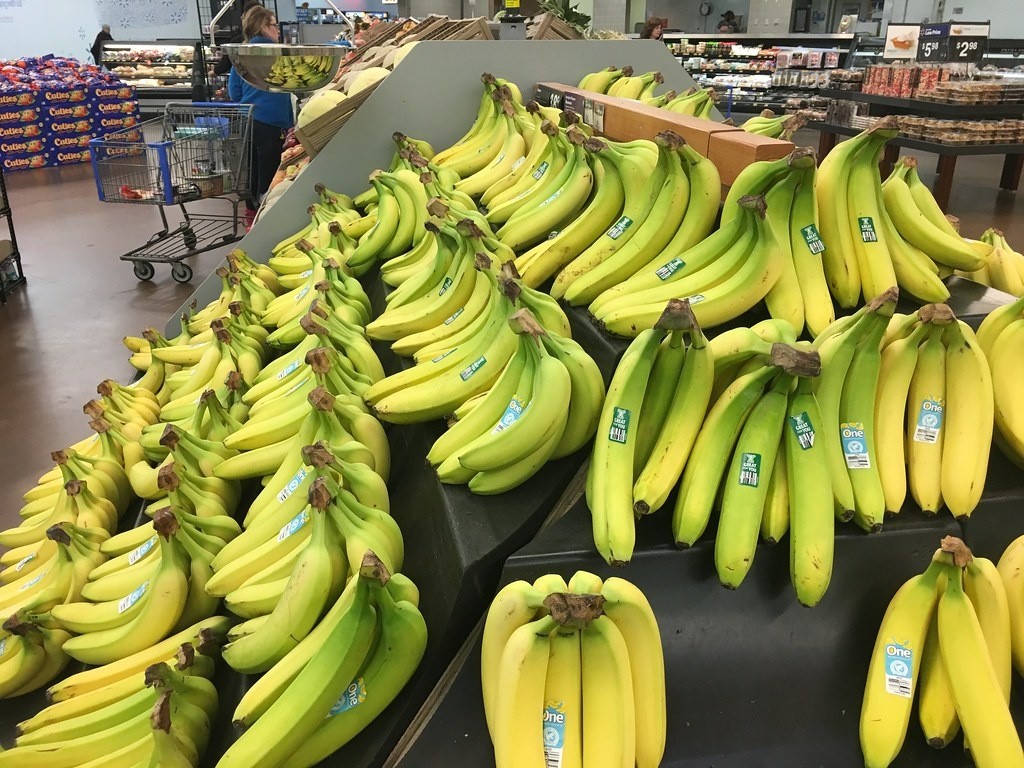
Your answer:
[700,1,710,16]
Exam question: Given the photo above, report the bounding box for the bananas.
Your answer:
[481,570,666,768]
[346,67,1024,606]
[859,534,1024,768]
[0,183,428,767]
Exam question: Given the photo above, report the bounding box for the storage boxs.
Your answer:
[357,11,581,58]
[0,88,143,172]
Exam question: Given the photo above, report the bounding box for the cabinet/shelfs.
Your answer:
[99,39,224,115]
[0,23,1023,767]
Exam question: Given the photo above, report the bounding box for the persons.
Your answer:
[227,5,295,234]
[718,10,739,34]
[91,24,114,65]
[639,17,664,42]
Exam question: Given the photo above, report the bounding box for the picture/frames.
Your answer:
[794,8,808,32]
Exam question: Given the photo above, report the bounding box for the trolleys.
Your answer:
[87,100,262,284]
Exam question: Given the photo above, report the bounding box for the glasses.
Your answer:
[267,23,279,28]
